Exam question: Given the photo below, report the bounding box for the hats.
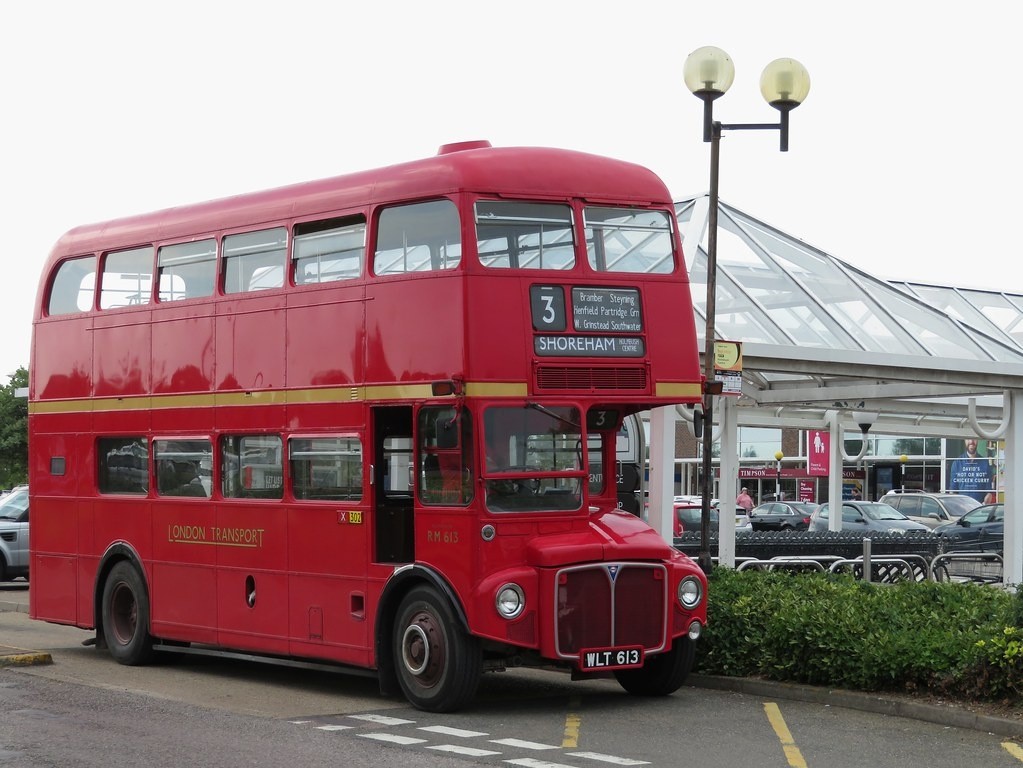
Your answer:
[742,487,748,491]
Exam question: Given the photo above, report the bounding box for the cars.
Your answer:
[929,503,1004,554]
[808,501,933,536]
[749,500,819,533]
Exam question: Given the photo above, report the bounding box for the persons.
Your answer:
[736,487,755,510]
[849,487,862,501]
[948,439,992,506]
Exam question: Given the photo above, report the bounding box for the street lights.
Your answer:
[774,451,784,502]
[681,45,810,572]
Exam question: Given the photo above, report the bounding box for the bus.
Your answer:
[28,139,708,714]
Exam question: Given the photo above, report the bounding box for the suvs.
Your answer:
[877,489,986,531]
[0,484,28,582]
[643,495,753,540]
